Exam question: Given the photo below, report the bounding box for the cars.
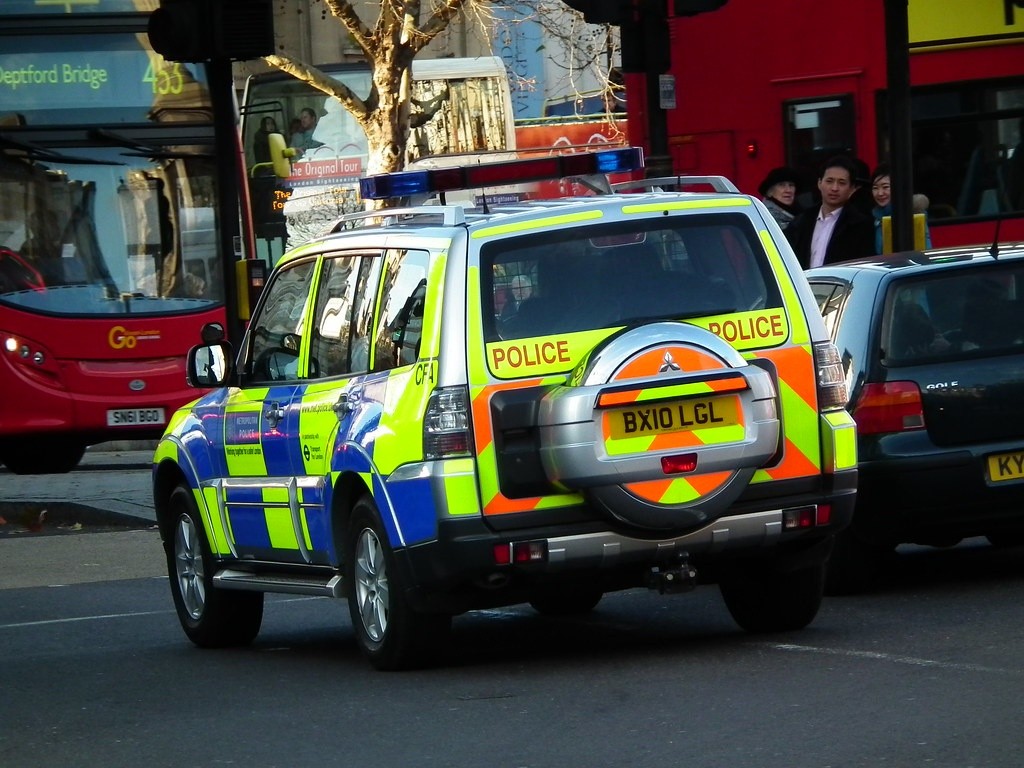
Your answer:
[751,240,1024,597]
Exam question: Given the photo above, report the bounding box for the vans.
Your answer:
[176,207,282,303]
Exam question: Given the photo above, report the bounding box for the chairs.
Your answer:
[512,244,734,336]
[892,277,1024,358]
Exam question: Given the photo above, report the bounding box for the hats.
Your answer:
[759,168,804,198]
[849,160,871,182]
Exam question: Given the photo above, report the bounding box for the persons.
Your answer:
[252,116,285,167]
[287,107,323,161]
[785,154,876,269]
[871,163,934,256]
[1009,120,1024,210]
[20,208,61,267]
[594,92,627,120]
[501,274,534,319]
[286,118,305,146]
[758,166,803,233]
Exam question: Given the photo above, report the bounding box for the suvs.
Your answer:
[151,145,860,660]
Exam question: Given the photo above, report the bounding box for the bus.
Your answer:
[1,1,295,475]
[237,54,516,326]
